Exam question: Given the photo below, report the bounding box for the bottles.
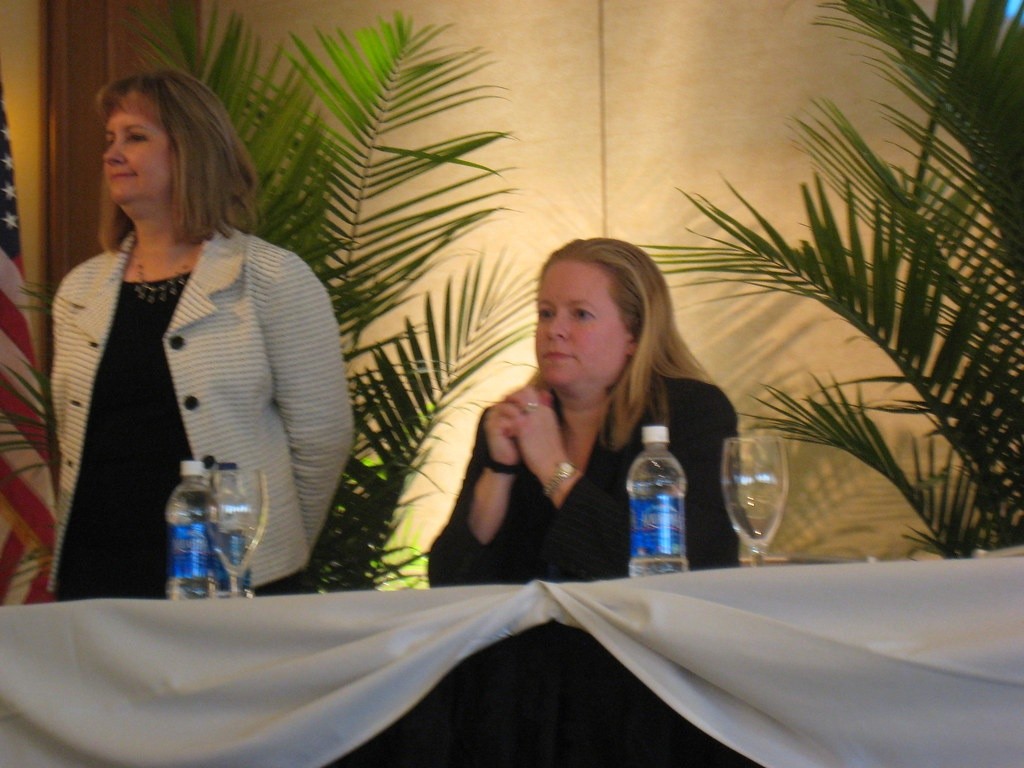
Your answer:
[165,459,213,602]
[626,425,689,577]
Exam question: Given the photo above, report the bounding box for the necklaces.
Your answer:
[131,231,192,304]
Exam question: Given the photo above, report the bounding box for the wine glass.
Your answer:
[206,469,269,599]
[721,437,790,567]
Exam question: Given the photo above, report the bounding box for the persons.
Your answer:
[430,237,741,585]
[50,70,354,597]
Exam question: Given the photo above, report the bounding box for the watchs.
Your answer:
[543,460,576,497]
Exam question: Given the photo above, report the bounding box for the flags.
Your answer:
[0,79,57,605]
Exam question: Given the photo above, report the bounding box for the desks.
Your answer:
[1,555,1024,768]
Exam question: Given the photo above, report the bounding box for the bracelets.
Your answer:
[485,459,522,475]
